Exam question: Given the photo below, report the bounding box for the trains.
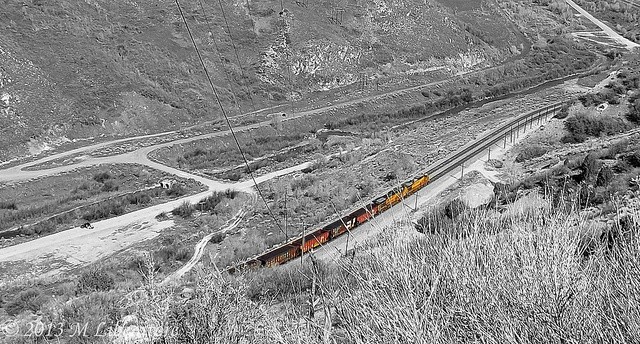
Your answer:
[227,172,430,277]
